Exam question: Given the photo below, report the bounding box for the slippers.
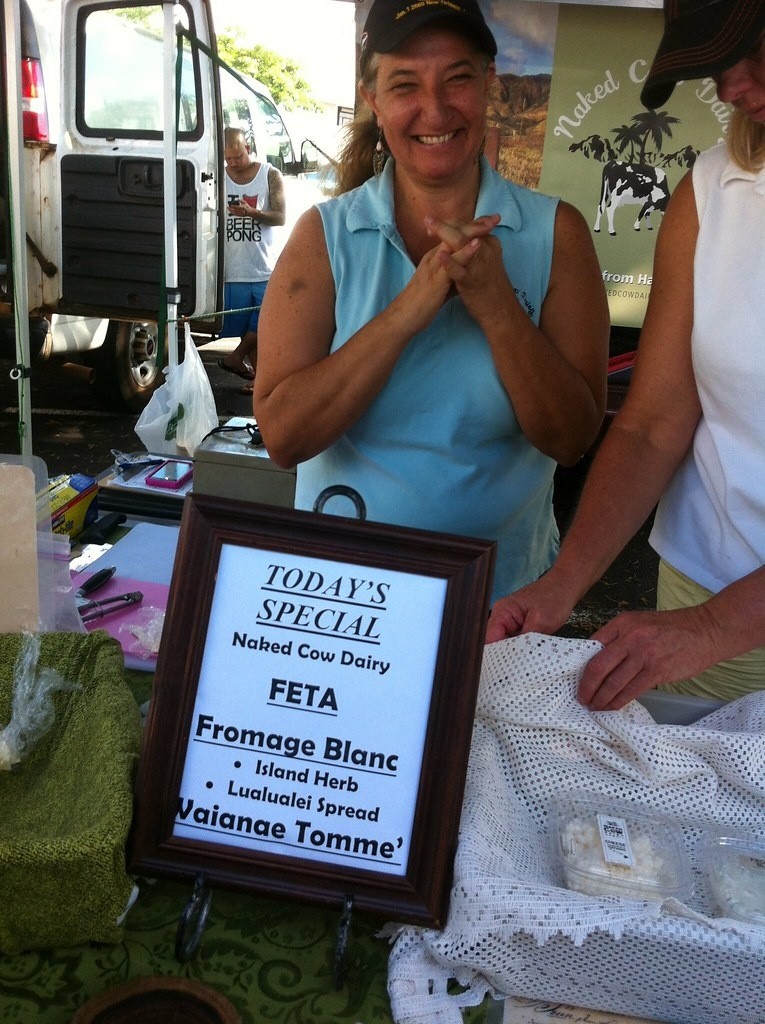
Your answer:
[219,358,255,379]
[241,379,254,394]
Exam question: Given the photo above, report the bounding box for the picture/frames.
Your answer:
[123,493,493,934]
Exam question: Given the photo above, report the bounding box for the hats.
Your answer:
[360,0,497,56]
[641,0,765,109]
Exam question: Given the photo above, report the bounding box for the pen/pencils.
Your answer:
[119,459,164,467]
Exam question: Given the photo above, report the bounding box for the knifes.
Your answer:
[74,566,116,606]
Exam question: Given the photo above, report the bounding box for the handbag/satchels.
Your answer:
[135,317,218,457]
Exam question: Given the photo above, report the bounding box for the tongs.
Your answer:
[77,591,143,624]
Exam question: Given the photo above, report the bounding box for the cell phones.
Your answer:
[145,459,194,490]
[228,201,240,207]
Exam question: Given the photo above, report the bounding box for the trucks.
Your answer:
[0,1,320,411]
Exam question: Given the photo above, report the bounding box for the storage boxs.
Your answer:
[549,789,696,904]
[702,828,765,926]
[465,690,765,1024]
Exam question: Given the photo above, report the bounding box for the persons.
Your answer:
[217,128,286,396]
[252,0,609,607]
[481,0,765,712]
